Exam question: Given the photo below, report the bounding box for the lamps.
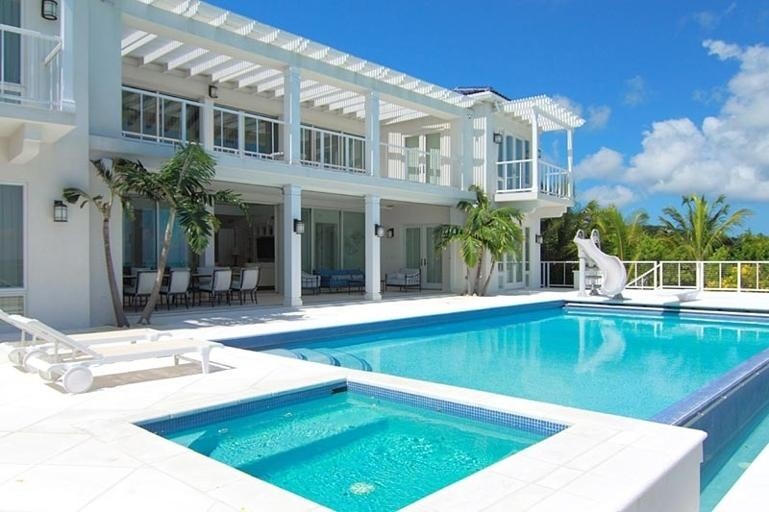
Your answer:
[40,0,59,22]
[375,224,385,237]
[493,133,503,145]
[294,219,305,234]
[53,199,68,222]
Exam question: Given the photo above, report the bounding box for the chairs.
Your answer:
[301,268,422,293]
[0,306,225,394]
[123,262,262,312]
